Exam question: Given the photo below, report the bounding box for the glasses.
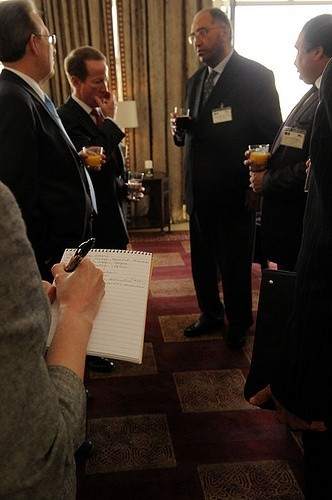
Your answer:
[186,25,224,45]
[35,33,57,46]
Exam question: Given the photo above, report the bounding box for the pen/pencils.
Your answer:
[48,238,97,304]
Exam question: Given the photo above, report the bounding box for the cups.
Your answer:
[83,146,104,171]
[174,106,190,133]
[249,144,269,172]
[127,171,144,202]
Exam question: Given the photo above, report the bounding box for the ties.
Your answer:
[270,86,317,152]
[200,70,219,110]
[91,108,105,129]
[44,93,98,213]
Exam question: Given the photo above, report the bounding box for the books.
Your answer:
[47,248,152,365]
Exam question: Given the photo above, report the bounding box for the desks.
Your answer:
[141,177,171,234]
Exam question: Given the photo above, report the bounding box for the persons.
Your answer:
[0,0,332,454]
[0,182,107,500]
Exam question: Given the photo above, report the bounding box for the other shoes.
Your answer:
[226,325,249,353]
[183,318,225,337]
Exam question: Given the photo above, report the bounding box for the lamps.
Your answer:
[117,101,139,170]
[145,160,153,177]
